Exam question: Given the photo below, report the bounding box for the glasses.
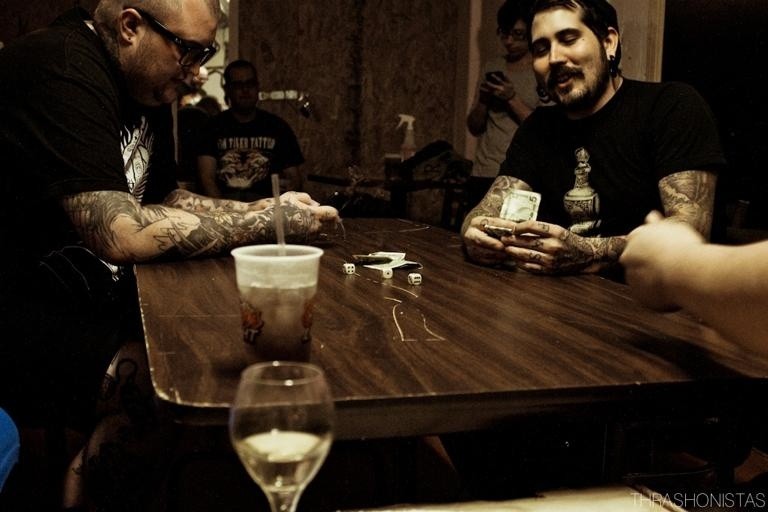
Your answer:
[496,26,527,40]
[122,6,217,67]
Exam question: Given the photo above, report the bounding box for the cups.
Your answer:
[231,244,324,368]
[384,153,402,181]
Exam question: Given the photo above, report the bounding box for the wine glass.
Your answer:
[229,360,338,512]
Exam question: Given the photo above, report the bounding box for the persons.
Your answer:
[457,0,722,504]
[465,0,554,215]
[196,56,307,205]
[155,94,222,196]
[0,0,341,512]
[615,207,767,359]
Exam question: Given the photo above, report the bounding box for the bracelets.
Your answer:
[504,91,516,103]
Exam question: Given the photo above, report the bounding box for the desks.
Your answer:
[307,174,447,219]
[63,218,768,508]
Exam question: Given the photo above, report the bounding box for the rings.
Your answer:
[317,232,328,241]
